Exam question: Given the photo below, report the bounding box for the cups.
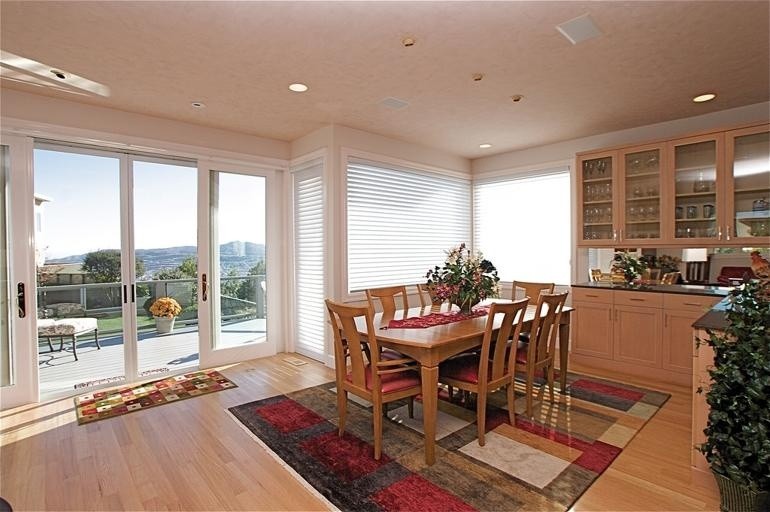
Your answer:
[675,205,716,238]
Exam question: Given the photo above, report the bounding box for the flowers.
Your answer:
[149,297,182,318]
[421,242,501,305]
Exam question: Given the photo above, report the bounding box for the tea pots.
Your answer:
[753,199,770,211]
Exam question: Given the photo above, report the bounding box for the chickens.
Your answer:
[750,250,770,288]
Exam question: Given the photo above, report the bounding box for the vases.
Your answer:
[452,288,483,313]
[154,315,175,333]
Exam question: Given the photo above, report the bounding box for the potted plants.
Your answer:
[692,281,770,511]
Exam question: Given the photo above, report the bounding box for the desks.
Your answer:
[326,298,577,466]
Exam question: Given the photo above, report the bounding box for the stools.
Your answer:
[38,316,100,362]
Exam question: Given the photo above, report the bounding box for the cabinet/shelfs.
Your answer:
[662,292,728,384]
[667,119,770,247]
[575,139,667,248]
[570,286,662,381]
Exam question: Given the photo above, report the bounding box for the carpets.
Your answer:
[224,364,672,512]
[72,366,239,426]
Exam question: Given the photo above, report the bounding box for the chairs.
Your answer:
[416,282,443,306]
[504,281,556,392]
[438,297,531,446]
[323,297,424,460]
[475,289,570,418]
[365,284,423,420]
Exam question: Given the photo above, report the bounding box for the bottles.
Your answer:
[693,169,710,192]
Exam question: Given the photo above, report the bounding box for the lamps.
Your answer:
[682,249,708,285]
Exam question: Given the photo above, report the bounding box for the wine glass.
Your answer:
[624,149,659,238]
[581,156,613,240]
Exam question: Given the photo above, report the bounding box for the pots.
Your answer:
[751,222,770,236]
